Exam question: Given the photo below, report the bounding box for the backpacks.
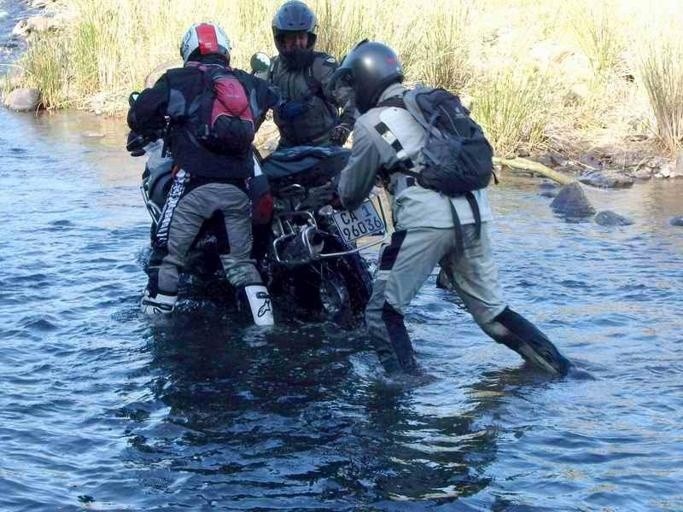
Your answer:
[378,84,492,192]
[183,60,256,155]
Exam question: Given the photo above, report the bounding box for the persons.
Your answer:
[127,22,276,330]
[254,1,358,148]
[324,42,581,383]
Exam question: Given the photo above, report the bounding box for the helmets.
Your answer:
[180,24,230,64]
[339,40,402,113]
[273,0,319,67]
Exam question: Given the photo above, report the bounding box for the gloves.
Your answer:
[331,122,354,146]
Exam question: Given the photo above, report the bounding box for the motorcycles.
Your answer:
[124,52,391,333]
